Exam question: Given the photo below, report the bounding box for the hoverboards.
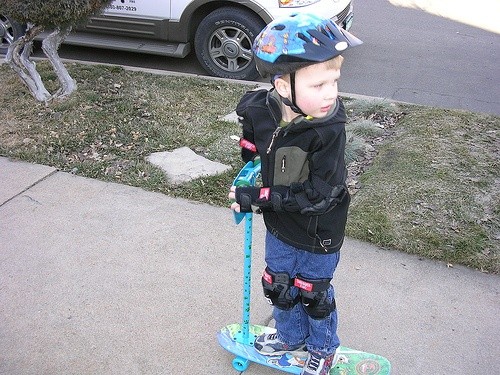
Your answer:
[218,153,393,374]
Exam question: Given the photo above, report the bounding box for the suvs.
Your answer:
[0,0,354,81]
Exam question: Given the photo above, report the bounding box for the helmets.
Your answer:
[253,10,364,78]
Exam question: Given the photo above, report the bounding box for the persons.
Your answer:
[228,11,363,375]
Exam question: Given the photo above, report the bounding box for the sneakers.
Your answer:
[254,333,306,355]
[301,348,339,375]
